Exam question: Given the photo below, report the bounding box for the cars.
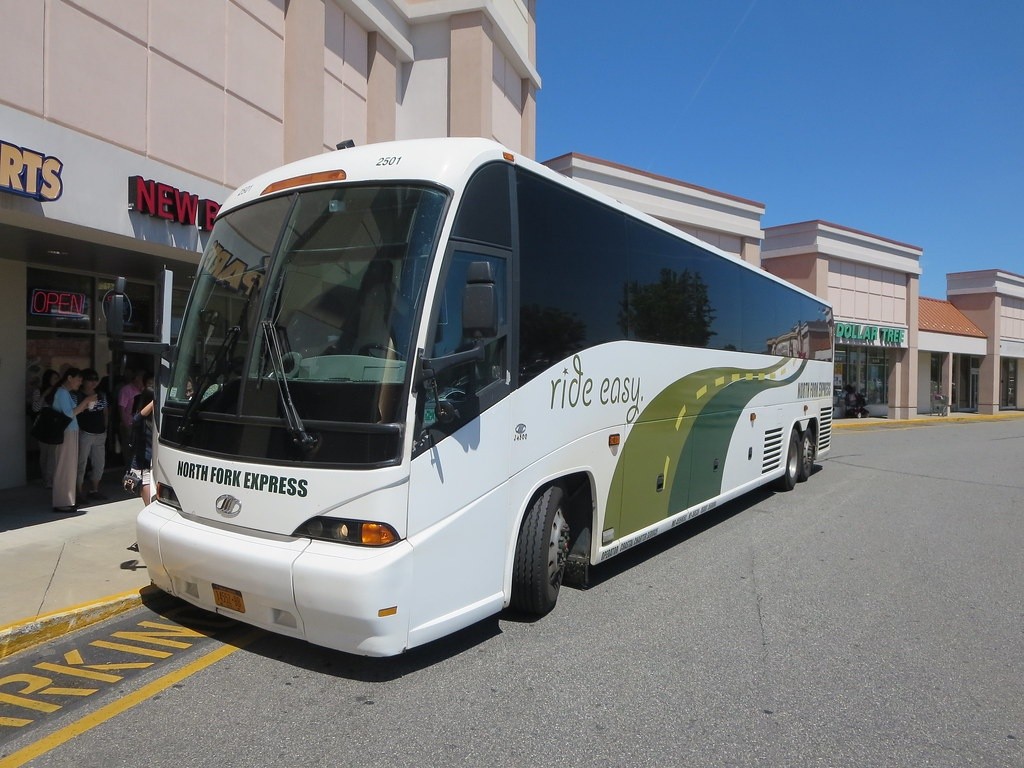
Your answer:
[852,374,883,391]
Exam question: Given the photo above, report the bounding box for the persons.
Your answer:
[73,369,108,506]
[96,360,253,509]
[49,366,98,512]
[30,366,61,489]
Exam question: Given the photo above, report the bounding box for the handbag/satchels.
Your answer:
[31,409,73,444]
[122,470,142,497]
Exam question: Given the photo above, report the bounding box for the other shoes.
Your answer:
[53,506,77,513]
[72,503,80,509]
[88,490,108,500]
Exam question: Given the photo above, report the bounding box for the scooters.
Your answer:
[845,391,869,418]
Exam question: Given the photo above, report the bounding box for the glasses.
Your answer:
[84,376,99,382]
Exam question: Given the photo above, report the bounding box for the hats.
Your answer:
[133,369,146,376]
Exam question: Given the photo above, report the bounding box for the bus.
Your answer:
[105,136,836,657]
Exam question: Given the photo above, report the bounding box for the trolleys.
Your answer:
[929,394,949,417]
